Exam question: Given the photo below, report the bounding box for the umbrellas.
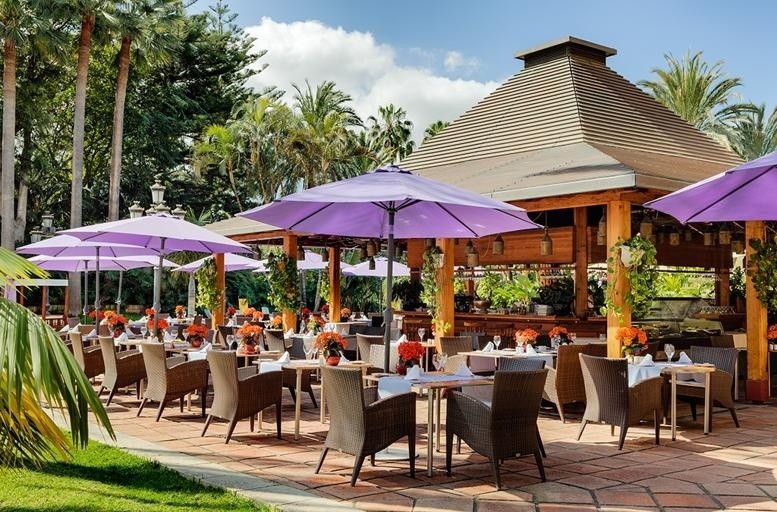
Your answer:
[225,164,546,372]
[640,148,777,225]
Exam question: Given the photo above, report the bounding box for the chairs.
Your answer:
[446,367,550,491]
[314,365,416,488]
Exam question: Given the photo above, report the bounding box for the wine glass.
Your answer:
[433,352,448,377]
[491,334,607,356]
[140,326,190,343]
[263,317,276,329]
[227,335,242,350]
[303,341,317,365]
[322,324,336,333]
[417,327,427,345]
[350,310,364,320]
[665,344,676,365]
